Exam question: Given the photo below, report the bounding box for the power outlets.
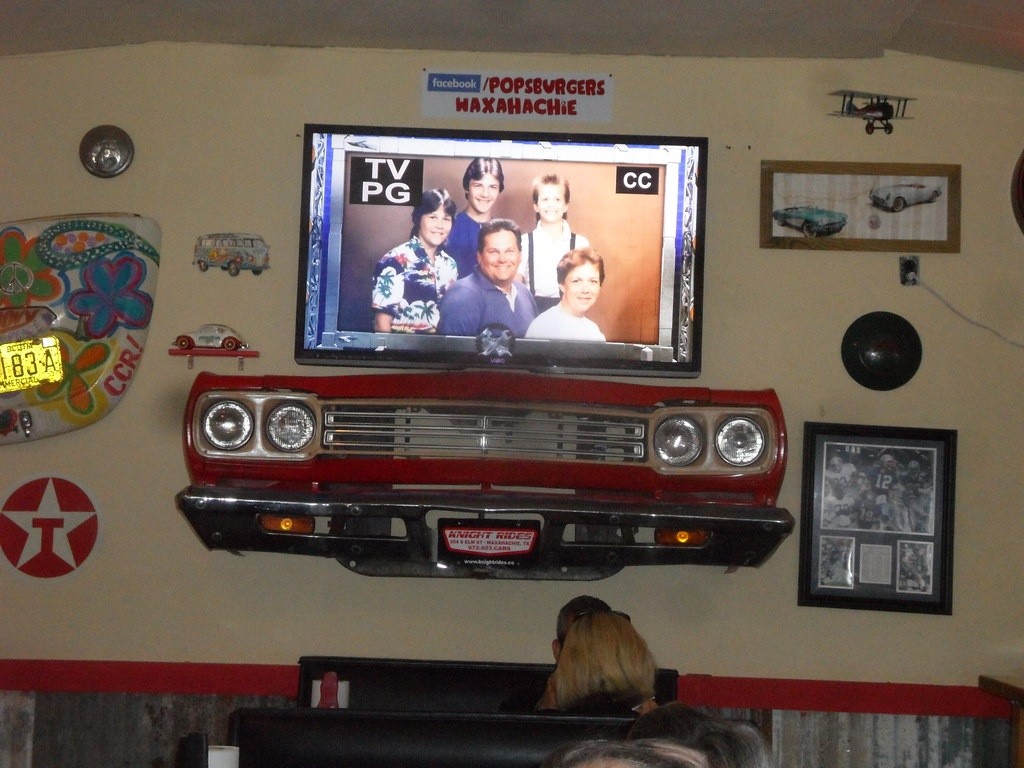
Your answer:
[899,254,918,285]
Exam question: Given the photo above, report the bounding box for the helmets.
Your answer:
[908,461,920,480]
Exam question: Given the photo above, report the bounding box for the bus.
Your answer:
[190,233,273,277]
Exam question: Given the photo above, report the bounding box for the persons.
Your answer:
[540,595,764,768]
[372,157,606,343]
[824,454,932,532]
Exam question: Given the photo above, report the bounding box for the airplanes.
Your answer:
[828,89,918,136]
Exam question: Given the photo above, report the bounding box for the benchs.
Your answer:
[230,656,681,768]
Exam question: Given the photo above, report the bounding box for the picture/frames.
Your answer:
[759,160,963,252]
[798,421,956,616]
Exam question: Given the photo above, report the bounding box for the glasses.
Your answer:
[575,610,630,622]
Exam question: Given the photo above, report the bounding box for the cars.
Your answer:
[174,325,247,349]
[773,206,849,239]
[868,180,941,212]
